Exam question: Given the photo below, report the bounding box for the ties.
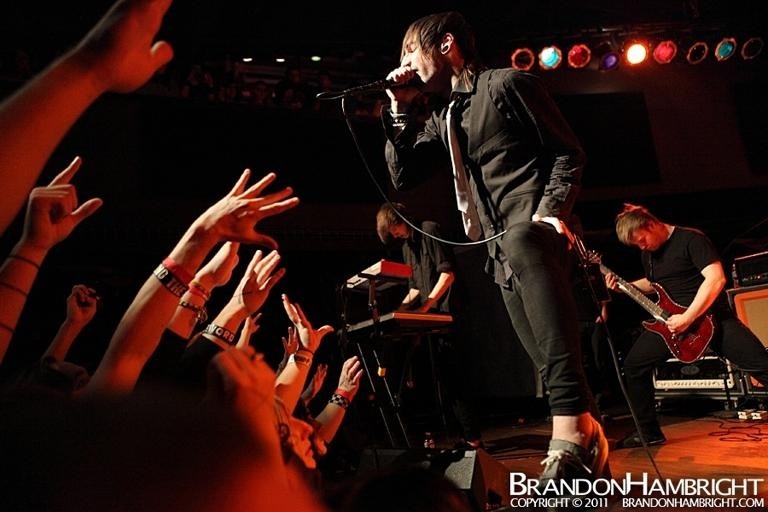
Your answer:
[446,100,482,240]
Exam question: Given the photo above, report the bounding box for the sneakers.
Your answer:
[614,428,666,448]
[534,418,608,505]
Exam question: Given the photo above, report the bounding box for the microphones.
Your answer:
[344,72,417,99]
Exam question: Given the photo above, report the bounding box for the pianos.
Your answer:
[334,310,457,341]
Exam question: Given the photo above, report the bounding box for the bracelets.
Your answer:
[390,112,406,127]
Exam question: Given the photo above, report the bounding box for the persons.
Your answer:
[374,202,484,450]
[604,202,767,447]
[379,13,612,509]
[1,1,365,511]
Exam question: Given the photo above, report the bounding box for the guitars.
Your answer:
[583,249,716,364]
